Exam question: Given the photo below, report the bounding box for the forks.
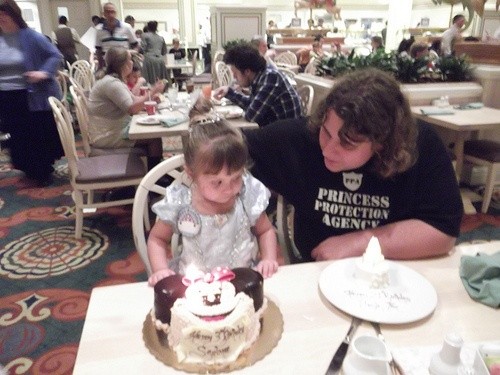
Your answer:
[370,322,407,375]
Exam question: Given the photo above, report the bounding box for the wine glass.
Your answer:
[185,78,194,107]
[166,87,178,111]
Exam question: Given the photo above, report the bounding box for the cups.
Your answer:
[139,87,149,110]
[342,335,398,374]
[143,101,157,116]
[202,83,212,99]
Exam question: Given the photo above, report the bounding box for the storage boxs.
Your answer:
[474,341,500,375]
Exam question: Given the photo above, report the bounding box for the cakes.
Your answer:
[151,266,269,365]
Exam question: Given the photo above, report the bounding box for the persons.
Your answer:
[265,19,278,47]
[462,34,480,45]
[427,39,443,67]
[94,2,140,71]
[0,0,65,189]
[233,68,465,264]
[146,94,279,288]
[381,21,388,47]
[483,24,500,44]
[138,20,169,94]
[212,39,306,142]
[118,61,164,162]
[298,14,358,68]
[368,37,387,58]
[51,14,82,79]
[397,36,416,65]
[409,43,435,73]
[440,15,467,59]
[91,14,107,72]
[170,18,210,44]
[134,28,144,48]
[168,36,186,91]
[87,45,165,201]
[248,37,271,55]
[122,13,137,41]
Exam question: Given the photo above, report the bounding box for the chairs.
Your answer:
[297,84,315,118]
[58,59,96,102]
[275,50,316,82]
[69,85,145,156]
[447,139,500,213]
[213,50,236,87]
[48,94,151,241]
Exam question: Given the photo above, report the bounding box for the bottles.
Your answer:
[430,337,465,374]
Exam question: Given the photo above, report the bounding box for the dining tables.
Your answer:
[72,242,500,375]
[166,62,193,91]
[409,105,500,203]
[129,91,261,140]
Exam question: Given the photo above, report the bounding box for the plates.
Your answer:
[318,257,438,324]
[475,343,499,375]
[137,116,164,124]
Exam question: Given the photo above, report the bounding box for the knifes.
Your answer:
[325,317,361,374]
